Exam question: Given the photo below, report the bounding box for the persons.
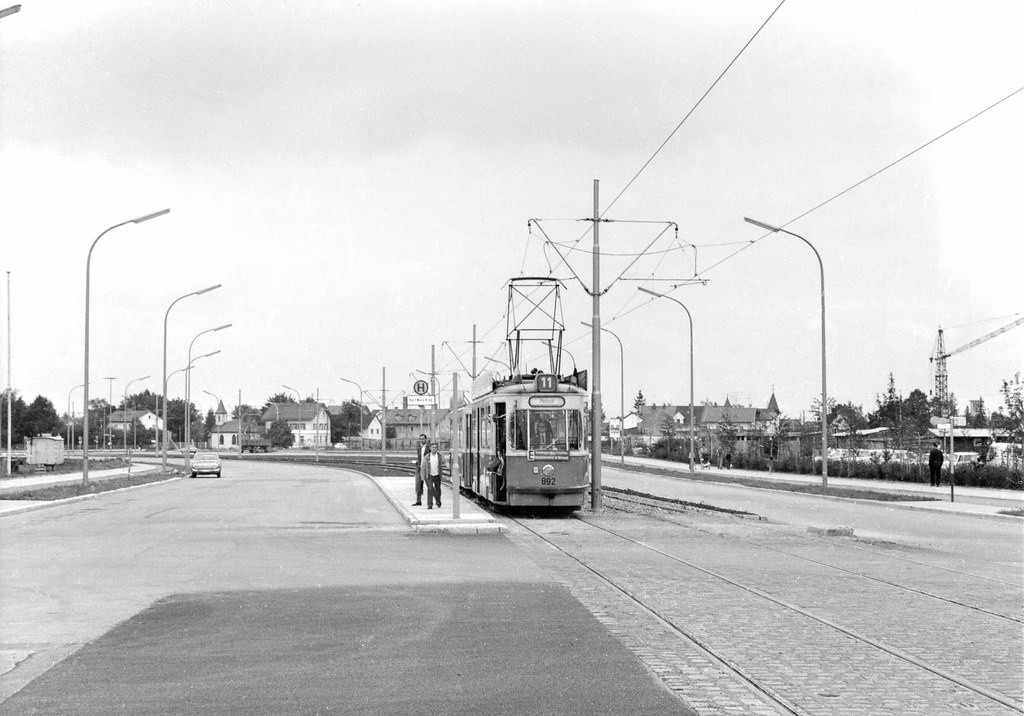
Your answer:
[531,421,558,450]
[497,414,525,494]
[688,451,695,470]
[928,442,944,487]
[726,450,732,469]
[411,434,443,509]
[716,444,724,470]
[698,443,709,467]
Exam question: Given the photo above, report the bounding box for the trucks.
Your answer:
[241,435,271,454]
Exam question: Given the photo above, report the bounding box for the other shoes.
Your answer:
[427,506,432,509]
[437,500,441,507]
[412,502,421,506]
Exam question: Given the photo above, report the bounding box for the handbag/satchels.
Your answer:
[487,457,501,472]
[730,463,733,468]
[497,451,504,476]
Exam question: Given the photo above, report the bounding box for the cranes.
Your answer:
[928,313,1024,423]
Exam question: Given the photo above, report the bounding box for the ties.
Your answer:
[542,434,544,443]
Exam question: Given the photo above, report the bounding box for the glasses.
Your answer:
[539,425,546,428]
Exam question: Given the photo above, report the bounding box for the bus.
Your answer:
[446,276,589,514]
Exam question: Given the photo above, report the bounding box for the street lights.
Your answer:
[163,282,222,481]
[202,390,220,453]
[124,375,152,458]
[165,323,233,468]
[67,383,92,452]
[264,400,279,421]
[580,320,625,467]
[637,287,696,474]
[340,377,364,453]
[82,208,172,490]
[542,341,576,381]
[282,385,302,449]
[743,216,828,489]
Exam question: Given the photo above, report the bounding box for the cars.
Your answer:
[941,451,977,469]
[191,453,222,479]
[180,445,197,453]
